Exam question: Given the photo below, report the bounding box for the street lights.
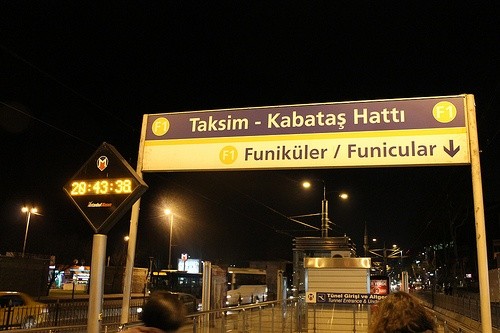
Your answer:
[166,207,174,270]
[300,180,348,238]
[20,206,37,254]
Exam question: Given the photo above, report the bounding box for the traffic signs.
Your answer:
[138,96,471,171]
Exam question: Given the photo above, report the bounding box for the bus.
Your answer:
[143,265,269,313]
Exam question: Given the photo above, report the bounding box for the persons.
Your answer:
[117,291,187,333]
[368,291,439,333]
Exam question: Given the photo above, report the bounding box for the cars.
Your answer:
[0,291,49,329]
